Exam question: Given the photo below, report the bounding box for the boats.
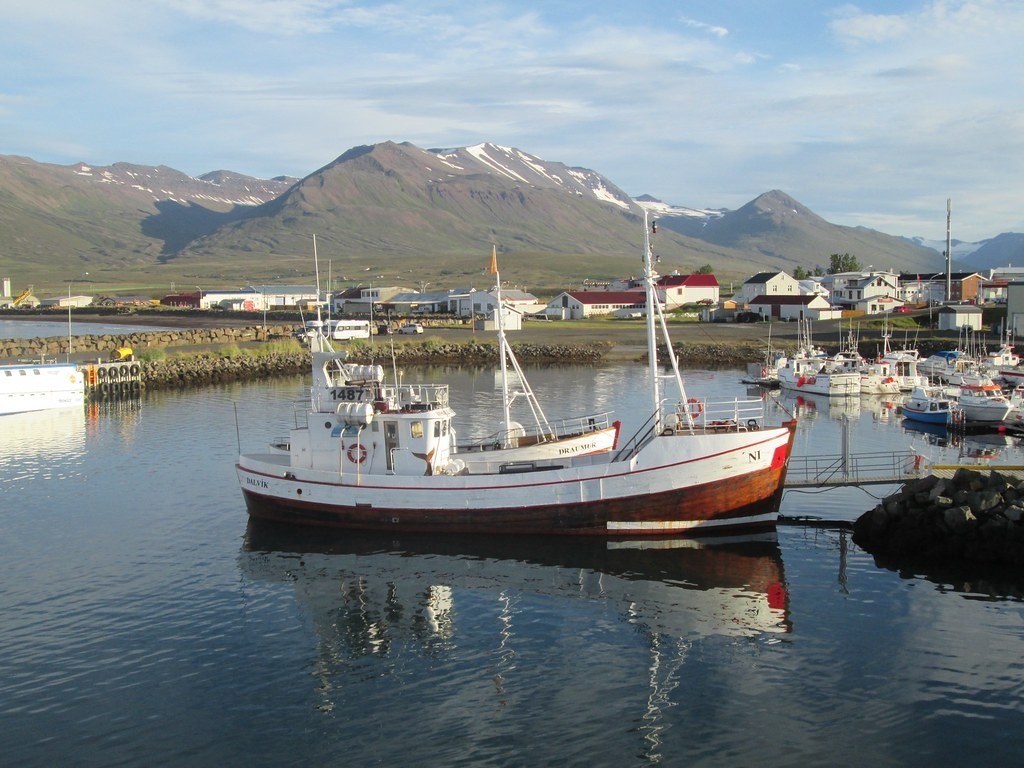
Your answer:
[231,197,803,540]
[236,514,795,768]
[741,315,1023,431]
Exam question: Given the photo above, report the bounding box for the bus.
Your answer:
[320,318,370,341]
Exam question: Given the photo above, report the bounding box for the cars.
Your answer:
[893,306,913,314]
[398,324,423,334]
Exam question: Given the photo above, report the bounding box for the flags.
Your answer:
[491,246,497,274]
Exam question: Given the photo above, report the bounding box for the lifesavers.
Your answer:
[952,410,964,424]
[761,368,767,378]
[682,397,703,418]
[347,443,367,464]
